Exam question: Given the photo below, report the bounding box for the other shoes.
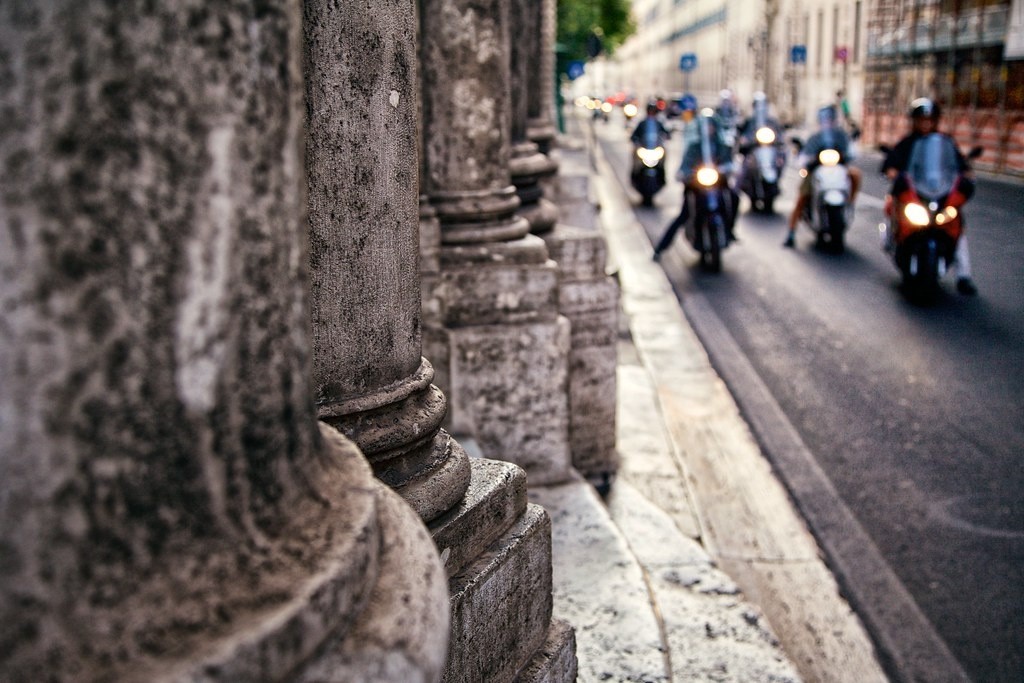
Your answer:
[728,233,737,242]
[956,275,978,297]
[783,237,794,246]
[653,252,660,261]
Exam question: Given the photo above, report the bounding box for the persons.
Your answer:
[879,96,979,301]
[627,103,672,186]
[780,104,861,249]
[736,92,786,180]
[713,88,739,138]
[652,109,742,262]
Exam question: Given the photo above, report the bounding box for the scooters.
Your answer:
[582,98,792,274]
[790,127,863,257]
[878,132,985,309]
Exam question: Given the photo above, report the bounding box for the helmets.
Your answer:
[697,109,719,134]
[906,98,938,119]
[647,103,658,111]
